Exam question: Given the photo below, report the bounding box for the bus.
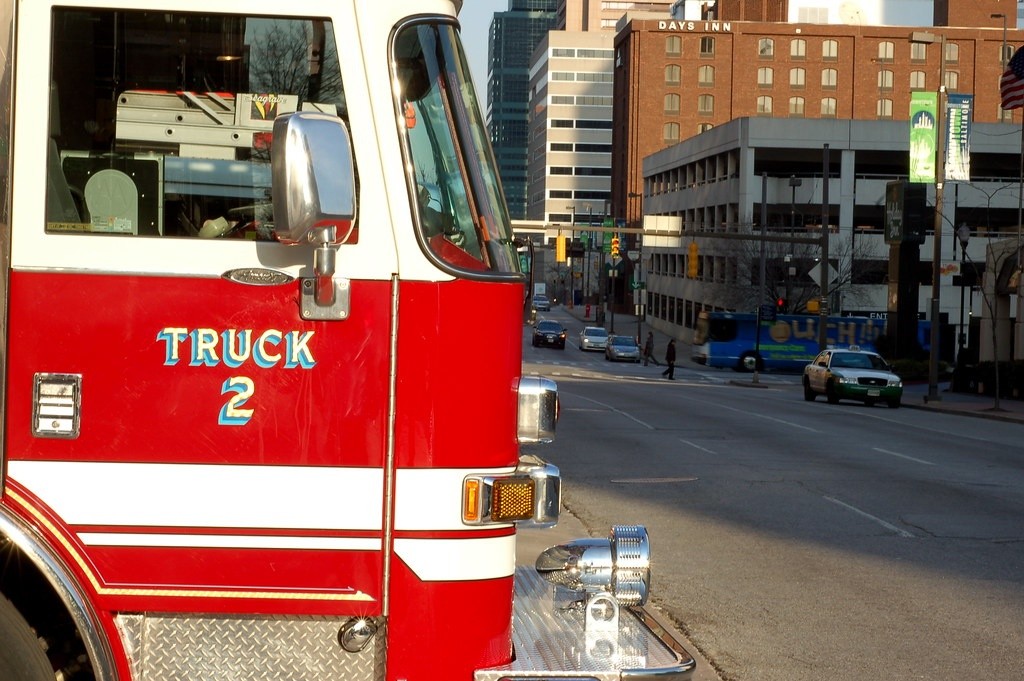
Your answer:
[690,311,941,373]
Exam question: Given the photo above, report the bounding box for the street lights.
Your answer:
[598,198,613,326]
[955,224,971,396]
[788,175,803,306]
[629,192,644,345]
[566,206,577,308]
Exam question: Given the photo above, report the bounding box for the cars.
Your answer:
[801,346,903,410]
[533,296,550,313]
[532,320,567,348]
[604,336,642,364]
[579,327,611,352]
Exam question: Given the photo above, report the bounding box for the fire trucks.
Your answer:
[1,1,699,681]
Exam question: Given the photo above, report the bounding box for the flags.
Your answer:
[1000,45,1024,110]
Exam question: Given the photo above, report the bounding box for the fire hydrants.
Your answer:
[584,303,590,319]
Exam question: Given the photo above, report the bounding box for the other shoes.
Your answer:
[643,365,648,367]
[662,372,667,378]
[668,376,675,380]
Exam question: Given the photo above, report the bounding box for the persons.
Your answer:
[662,337,677,380]
[643,331,661,368]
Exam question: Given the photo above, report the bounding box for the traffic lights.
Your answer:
[610,236,621,260]
[779,298,788,313]
